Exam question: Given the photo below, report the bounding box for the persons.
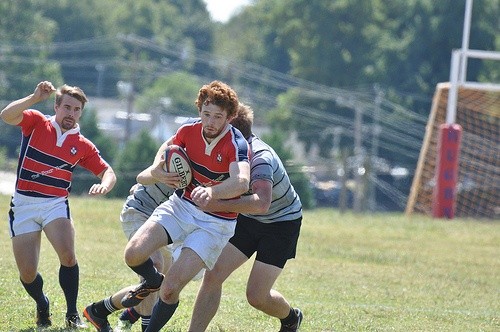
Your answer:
[83,164,177,332]
[0,81,117,328]
[121,81,251,332]
[189,102,303,332]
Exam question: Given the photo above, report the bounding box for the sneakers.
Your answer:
[65,311,80,329]
[121,273,165,308]
[83,303,115,332]
[279,308,303,332]
[35,297,51,329]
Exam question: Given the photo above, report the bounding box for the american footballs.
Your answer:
[159,145,192,188]
